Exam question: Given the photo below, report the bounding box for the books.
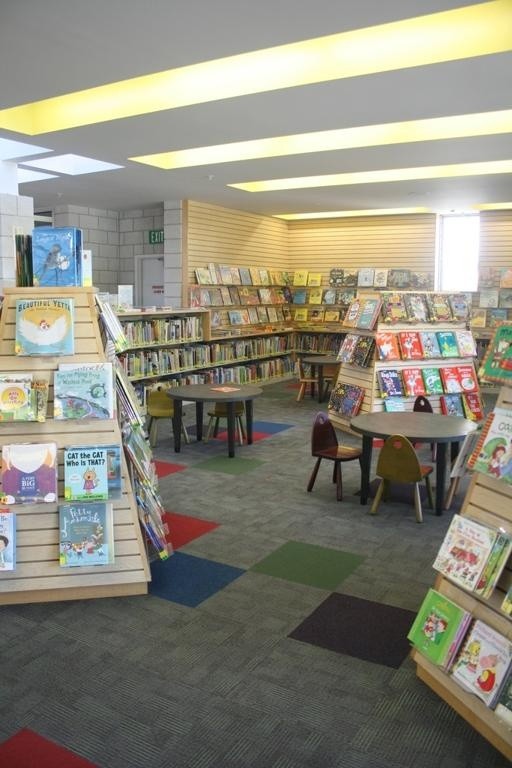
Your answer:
[1,225,168,570]
[407,320,512,726]
[111,262,512,426]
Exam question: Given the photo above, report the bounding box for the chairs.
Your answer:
[144,385,192,448]
[409,393,445,464]
[202,397,251,449]
[302,410,368,503]
[368,430,436,527]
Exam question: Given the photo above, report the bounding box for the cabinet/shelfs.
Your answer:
[0,280,175,612]
[102,260,297,406]
[289,282,512,439]
[400,320,511,768]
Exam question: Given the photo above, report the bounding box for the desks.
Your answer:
[167,379,262,455]
[349,409,478,518]
